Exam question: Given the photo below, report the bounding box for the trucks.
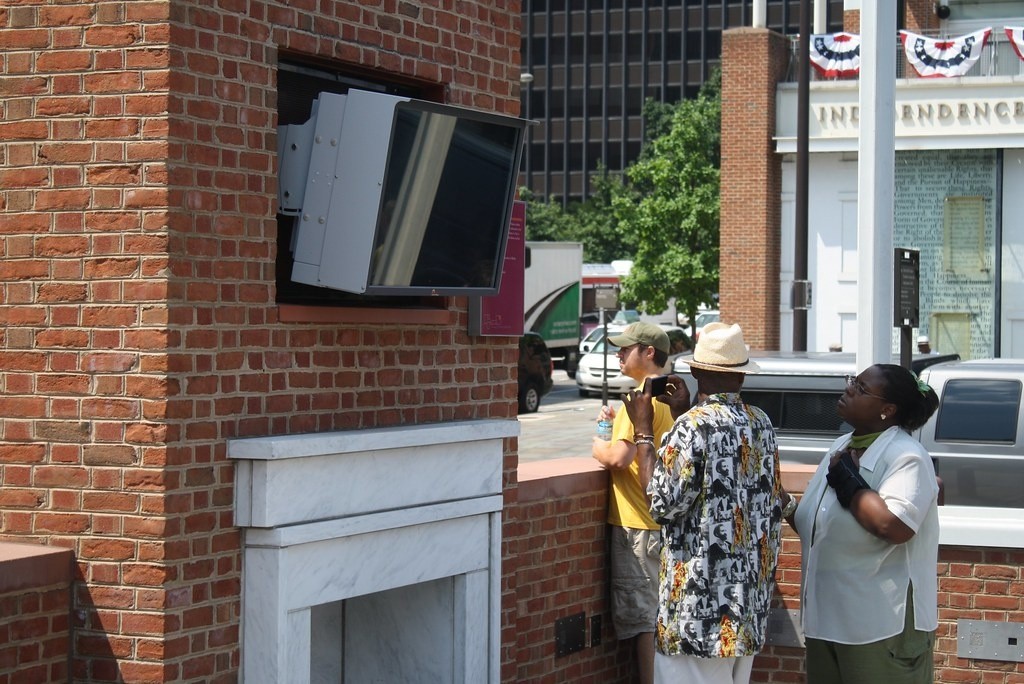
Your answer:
[524,242,585,355]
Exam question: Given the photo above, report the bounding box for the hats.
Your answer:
[682,322,761,376]
[606,322,670,355]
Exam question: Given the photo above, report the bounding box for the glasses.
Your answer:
[845,374,894,402]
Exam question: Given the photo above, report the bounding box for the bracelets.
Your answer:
[633,433,655,439]
[636,440,656,449]
[782,493,797,518]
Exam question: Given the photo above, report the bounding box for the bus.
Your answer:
[578,260,673,325]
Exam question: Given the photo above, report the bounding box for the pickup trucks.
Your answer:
[667,346,1023,508]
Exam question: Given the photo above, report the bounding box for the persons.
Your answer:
[592,320,778,684]
[780,364,940,684]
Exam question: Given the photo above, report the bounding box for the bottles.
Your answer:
[596,405,612,443]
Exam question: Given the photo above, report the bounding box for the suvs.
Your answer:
[517,332,553,415]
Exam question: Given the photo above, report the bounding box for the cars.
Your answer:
[578,309,720,402]
